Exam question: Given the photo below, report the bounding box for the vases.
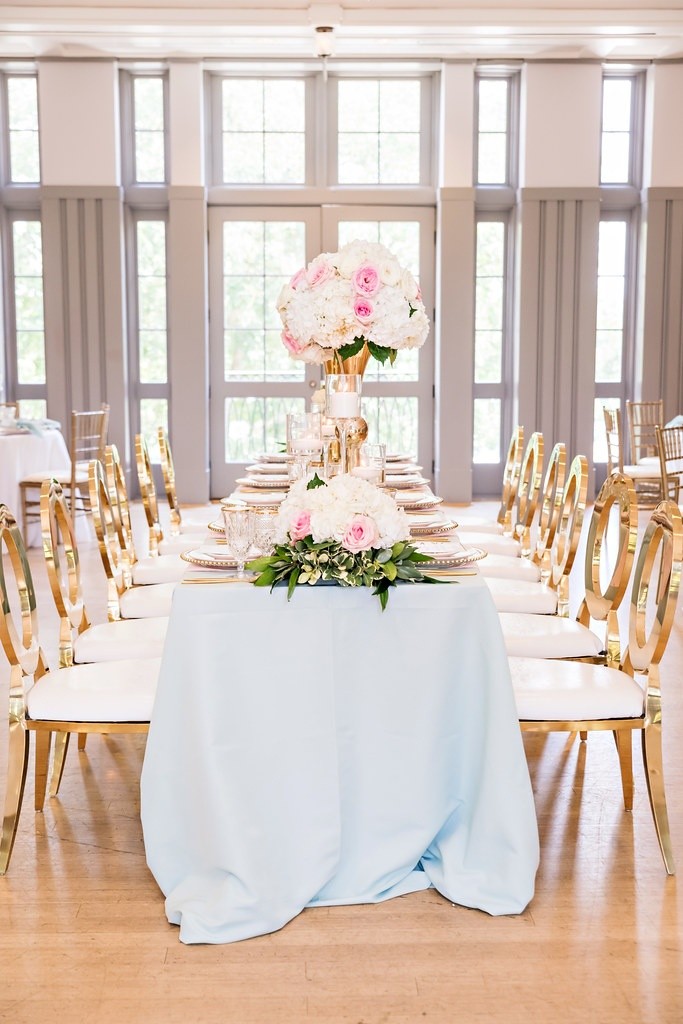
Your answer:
[324,345,373,474]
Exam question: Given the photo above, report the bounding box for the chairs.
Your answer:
[0,403,223,876]
[445,400,683,875]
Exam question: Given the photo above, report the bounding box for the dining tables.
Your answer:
[140,453,540,946]
[0,429,72,554]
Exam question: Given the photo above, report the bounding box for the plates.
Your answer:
[180,449,290,569]
[382,452,487,568]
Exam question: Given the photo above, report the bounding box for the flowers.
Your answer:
[244,471,458,614]
[274,240,430,365]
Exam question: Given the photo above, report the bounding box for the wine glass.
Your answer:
[221,506,256,581]
[286,411,322,479]
[325,374,363,475]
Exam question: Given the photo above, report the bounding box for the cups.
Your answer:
[350,443,385,483]
[252,505,279,558]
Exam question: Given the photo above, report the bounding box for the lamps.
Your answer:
[304,1,346,83]
